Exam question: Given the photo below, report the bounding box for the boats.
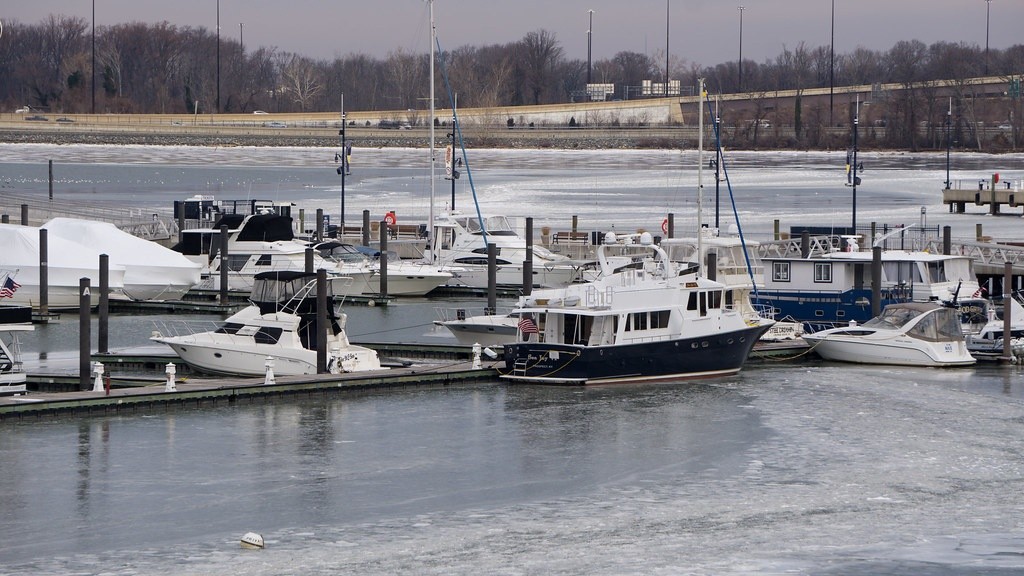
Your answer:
[800,303,977,368]
[431,315,812,361]
[171,213,454,296]
[0,304,32,396]
[422,215,700,299]
[432,232,777,387]
[149,271,392,378]
[748,249,994,335]
[963,320,1024,360]
[291,220,427,259]
[653,236,765,292]
[173,195,294,241]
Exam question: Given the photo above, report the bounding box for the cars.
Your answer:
[254,110,268,114]
[998,124,1012,129]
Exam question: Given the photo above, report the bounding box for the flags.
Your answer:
[0,276,21,299]
[972,284,987,297]
[518,318,539,334]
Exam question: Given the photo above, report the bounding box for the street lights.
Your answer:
[445,93,462,211]
[847,94,863,234]
[985,0,993,77]
[335,92,352,234]
[737,5,746,93]
[586,9,596,83]
[709,97,723,236]
[944,106,952,190]
[238,23,246,62]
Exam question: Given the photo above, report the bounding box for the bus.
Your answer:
[745,118,770,127]
